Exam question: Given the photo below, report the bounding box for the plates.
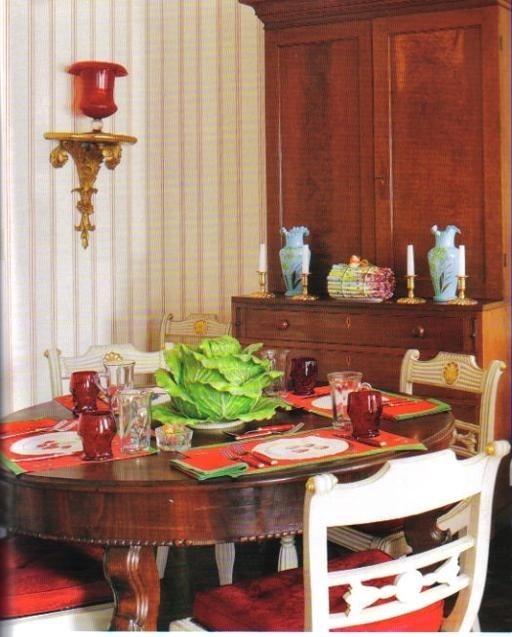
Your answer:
[251,437,349,460]
[311,392,389,409]
[9,431,83,454]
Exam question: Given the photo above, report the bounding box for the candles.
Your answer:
[259,243,267,272]
[457,244,466,278]
[407,243,415,277]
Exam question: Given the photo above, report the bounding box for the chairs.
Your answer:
[159,314,232,352]
[399,345,507,459]
[169,438,512,633]
[0,538,117,636]
[46,343,175,397]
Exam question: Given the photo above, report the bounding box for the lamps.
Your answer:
[45,62,138,250]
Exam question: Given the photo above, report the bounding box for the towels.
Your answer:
[171,454,249,481]
[382,396,451,420]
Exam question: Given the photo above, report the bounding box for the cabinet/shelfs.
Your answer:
[232,295,512,440]
[241,0,512,302]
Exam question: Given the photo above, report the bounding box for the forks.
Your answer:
[1,421,78,440]
[221,449,265,468]
[224,423,294,437]
[231,443,282,466]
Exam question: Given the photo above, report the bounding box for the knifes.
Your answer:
[235,423,308,440]
[333,432,388,447]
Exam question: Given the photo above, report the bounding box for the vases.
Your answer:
[280,225,312,297]
[428,222,462,301]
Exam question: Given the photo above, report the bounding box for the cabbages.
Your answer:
[150,335,293,426]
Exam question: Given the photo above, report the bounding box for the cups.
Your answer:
[327,371,372,432]
[97,360,137,408]
[347,391,382,438]
[70,371,101,415]
[292,357,319,394]
[262,348,289,393]
[79,410,116,459]
[118,388,152,454]
